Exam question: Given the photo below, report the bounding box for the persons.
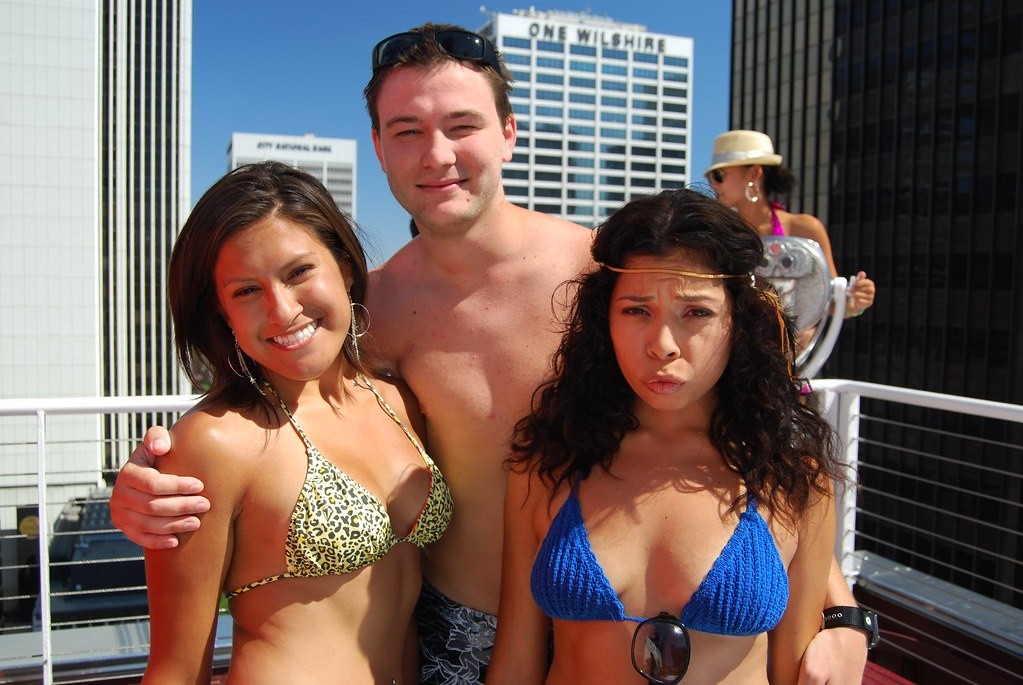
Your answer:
[484,181,863,685]
[705,130,876,449]
[139,160,455,685]
[109,22,869,685]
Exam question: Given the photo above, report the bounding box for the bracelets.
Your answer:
[846,306,865,317]
[820,606,880,650]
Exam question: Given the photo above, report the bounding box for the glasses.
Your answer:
[370,29,504,77]
[707,167,741,185]
[630,611,690,685]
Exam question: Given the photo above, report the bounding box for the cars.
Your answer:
[33,493,151,632]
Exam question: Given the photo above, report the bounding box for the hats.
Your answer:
[703,130,782,178]
